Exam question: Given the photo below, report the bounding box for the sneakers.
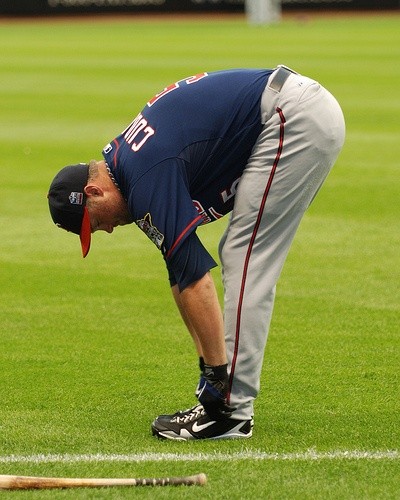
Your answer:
[151,403,255,441]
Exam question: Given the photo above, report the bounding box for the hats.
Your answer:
[46,163,92,257]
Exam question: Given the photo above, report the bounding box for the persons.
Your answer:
[46,64,348,443]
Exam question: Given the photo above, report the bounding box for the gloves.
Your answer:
[195,356,237,424]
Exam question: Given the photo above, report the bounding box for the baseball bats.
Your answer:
[0,473,207,488]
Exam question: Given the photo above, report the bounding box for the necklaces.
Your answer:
[105,162,121,192]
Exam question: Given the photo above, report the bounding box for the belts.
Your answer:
[269,67,291,92]
